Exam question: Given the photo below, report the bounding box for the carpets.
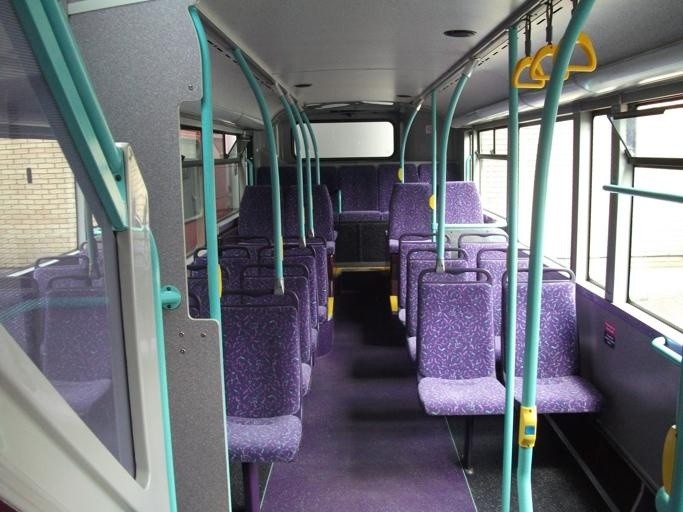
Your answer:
[259,289,476,511]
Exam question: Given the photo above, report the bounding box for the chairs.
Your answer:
[387,181,606,416]
[256,162,455,224]
[184,183,339,463]
